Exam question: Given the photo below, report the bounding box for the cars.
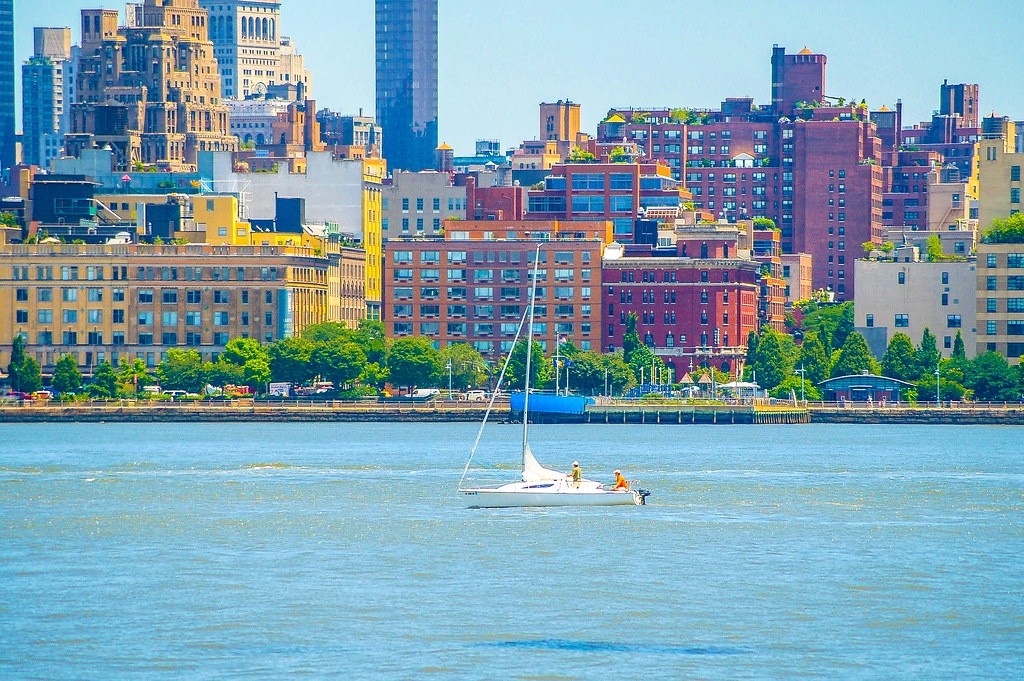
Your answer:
[161,390,187,397]
[458,390,485,402]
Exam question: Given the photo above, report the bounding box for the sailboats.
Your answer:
[455,242,651,511]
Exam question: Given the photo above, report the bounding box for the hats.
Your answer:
[571,460,579,466]
[614,469,620,473]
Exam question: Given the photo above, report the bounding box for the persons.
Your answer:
[612,470,628,491]
[566,461,581,482]
